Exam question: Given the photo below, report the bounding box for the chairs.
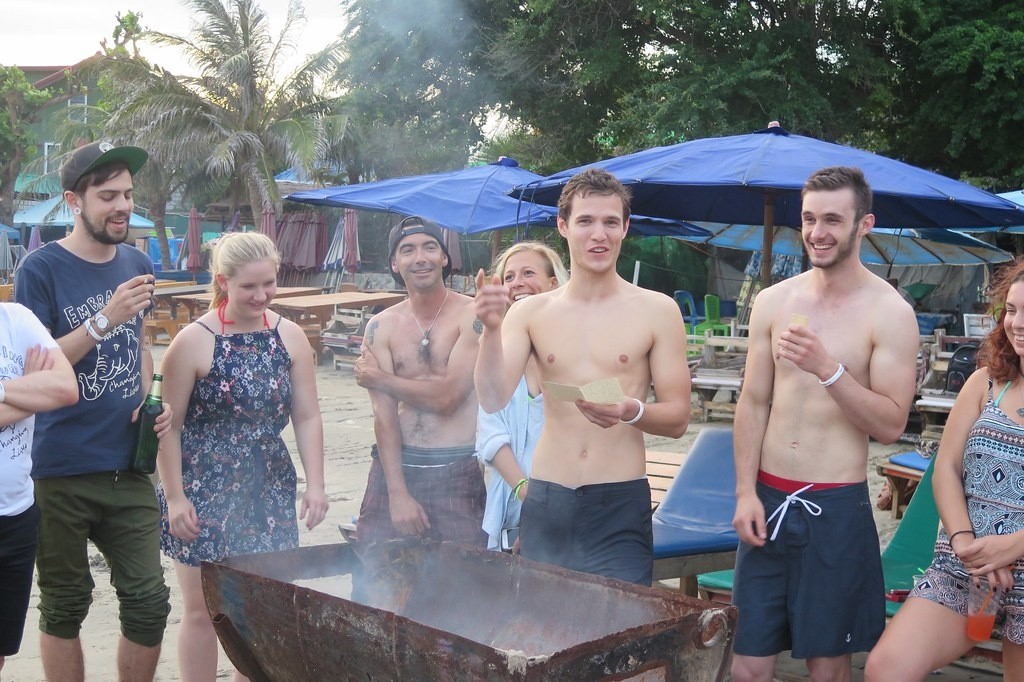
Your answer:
[702,451,942,621]
[673,297,704,356]
[674,290,705,343]
[645,449,689,510]
[651,427,740,598]
[694,294,731,352]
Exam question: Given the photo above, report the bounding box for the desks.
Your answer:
[267,291,407,335]
[169,286,323,323]
[149,284,210,321]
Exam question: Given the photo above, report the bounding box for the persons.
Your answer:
[12,142,173,682]
[0,301,79,682]
[472,166,691,589]
[473,241,567,556]
[152,232,329,682]
[862,260,1024,682]
[729,167,919,682]
[355,214,488,547]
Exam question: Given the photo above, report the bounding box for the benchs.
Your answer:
[148,304,377,369]
[899,328,986,447]
[876,450,932,520]
[689,318,751,424]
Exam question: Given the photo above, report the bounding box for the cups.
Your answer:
[967,578,1002,643]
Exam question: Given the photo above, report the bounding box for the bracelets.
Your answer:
[0,382,5,403]
[619,399,644,425]
[513,478,528,499]
[819,363,845,387]
[85,319,103,341]
[950,530,976,547]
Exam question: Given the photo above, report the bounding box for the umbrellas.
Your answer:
[0,191,175,286]
[179,203,361,296]
[287,121,1024,290]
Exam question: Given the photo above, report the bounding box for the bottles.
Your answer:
[129,375,163,474]
[977,285,991,303]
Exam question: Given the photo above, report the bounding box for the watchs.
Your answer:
[95,310,111,333]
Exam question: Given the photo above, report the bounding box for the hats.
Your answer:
[388,216,452,287]
[61,140,148,192]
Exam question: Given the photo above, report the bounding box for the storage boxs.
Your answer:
[964,313,993,338]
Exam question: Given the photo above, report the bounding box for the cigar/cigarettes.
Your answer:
[143,278,149,284]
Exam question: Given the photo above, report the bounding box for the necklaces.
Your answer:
[411,290,449,346]
